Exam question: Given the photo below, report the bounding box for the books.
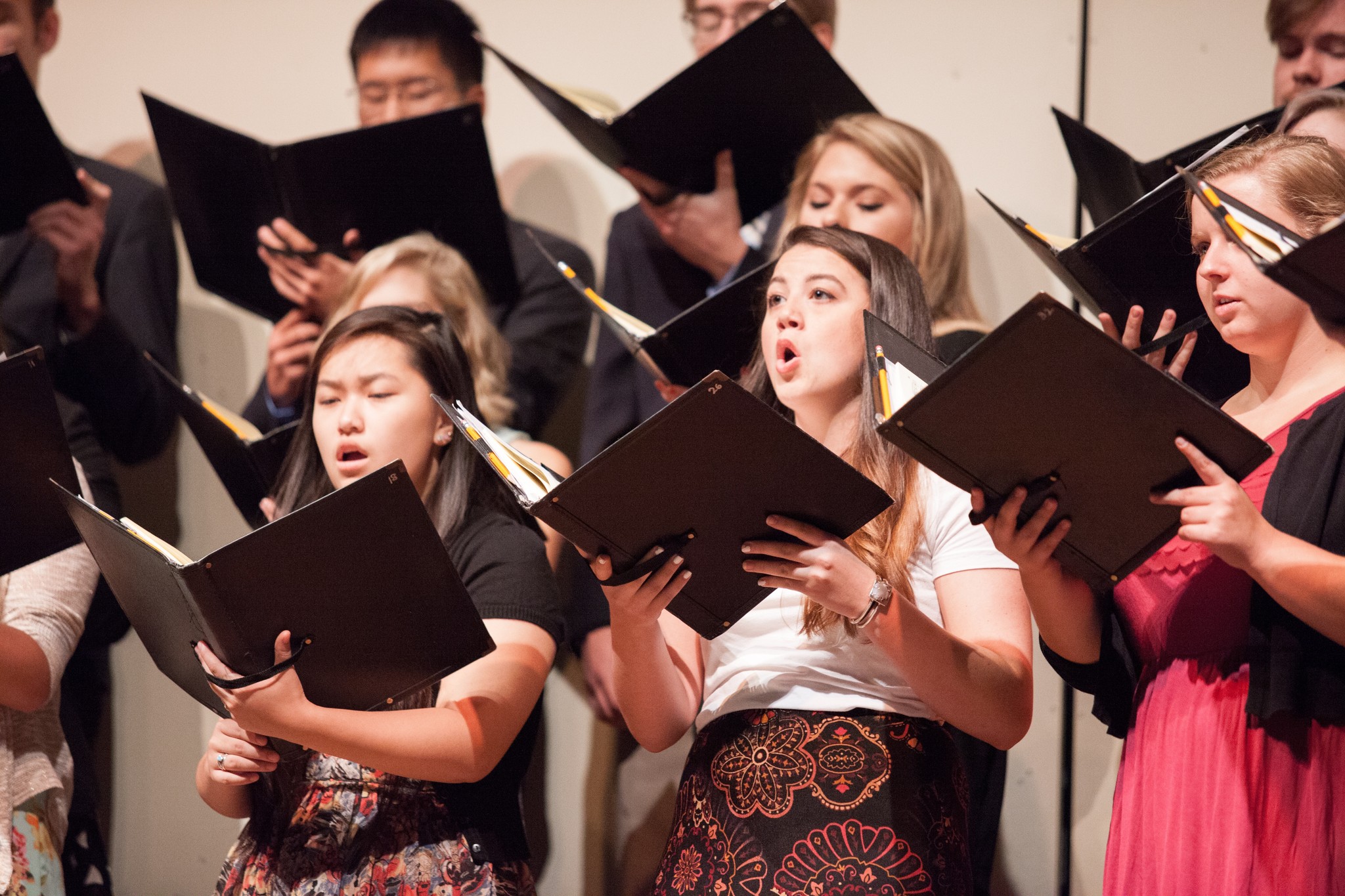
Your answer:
[974,122,1276,400]
[1050,78,1345,227]
[428,370,897,637]
[135,347,307,535]
[470,5,883,230]
[39,458,498,760]
[848,288,1267,594]
[0,344,88,578]
[132,87,522,327]
[529,239,779,386]
[1,52,86,236]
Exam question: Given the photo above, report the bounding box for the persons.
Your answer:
[0,382,102,895]
[971,131,1345,896]
[242,0,587,438]
[649,113,991,399]
[581,0,841,896]
[0,0,180,896]
[255,226,571,555]
[192,305,554,896]
[1265,0,1344,110]
[1098,85,1345,380]
[594,225,1033,894]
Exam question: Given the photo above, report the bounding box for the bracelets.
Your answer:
[850,573,892,628]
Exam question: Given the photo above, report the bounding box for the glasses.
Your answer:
[682,1,770,32]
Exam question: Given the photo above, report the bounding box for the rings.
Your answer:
[217,753,227,770]
